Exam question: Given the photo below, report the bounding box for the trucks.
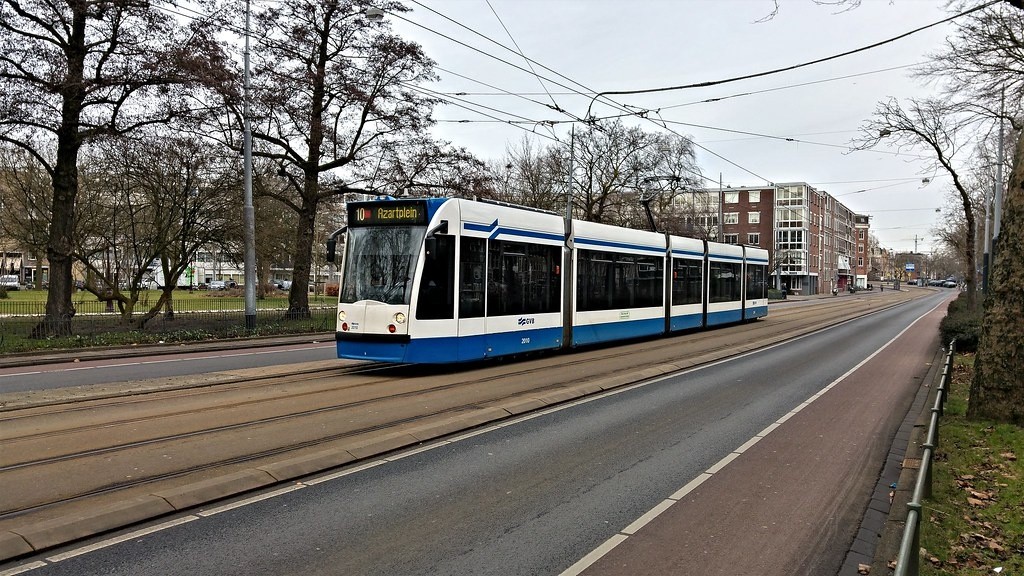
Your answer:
[143,264,199,290]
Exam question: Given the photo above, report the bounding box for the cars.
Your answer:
[268,279,292,291]
[74,280,128,291]
[191,281,245,291]
[906,277,957,288]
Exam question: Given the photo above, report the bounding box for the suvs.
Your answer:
[0,275,21,291]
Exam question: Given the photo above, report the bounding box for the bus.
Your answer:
[325,177,769,365]
